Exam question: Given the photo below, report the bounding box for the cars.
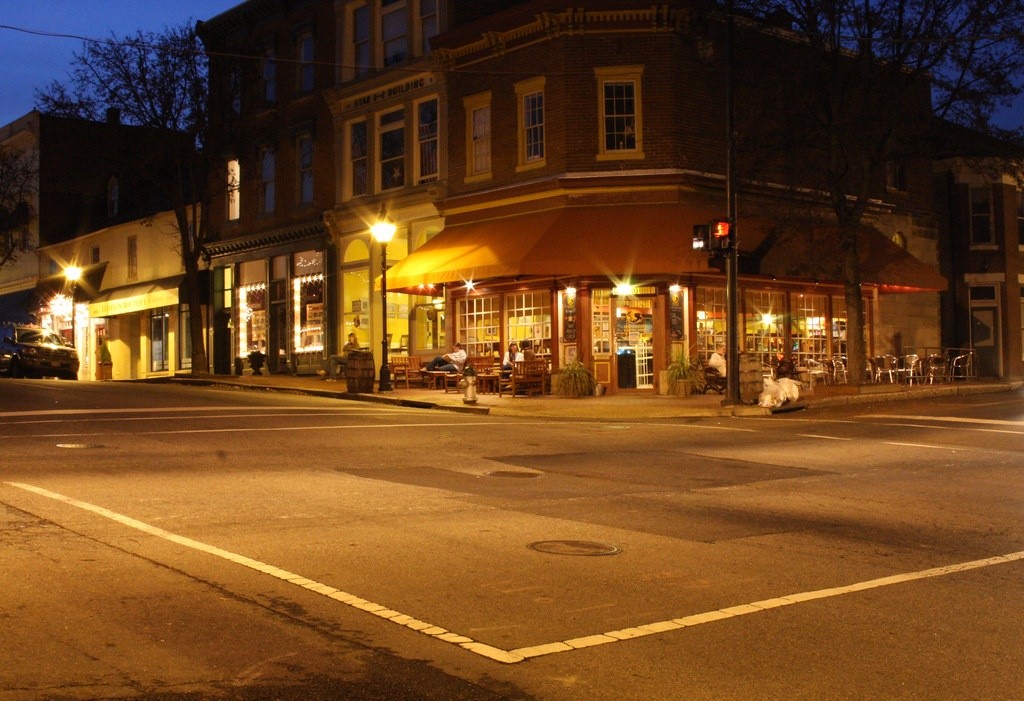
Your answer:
[1,329,80,380]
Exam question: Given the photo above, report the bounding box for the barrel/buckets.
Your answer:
[346,353,376,394]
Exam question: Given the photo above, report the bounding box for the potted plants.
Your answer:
[558,348,597,397]
[665,343,704,399]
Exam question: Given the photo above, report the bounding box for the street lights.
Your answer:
[64,264,81,349]
[371,203,396,392]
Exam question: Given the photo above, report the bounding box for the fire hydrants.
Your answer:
[458,365,480,403]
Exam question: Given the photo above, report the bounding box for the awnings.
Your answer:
[373,199,948,295]
[88,271,187,321]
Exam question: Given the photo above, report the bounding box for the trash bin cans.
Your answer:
[345,348,376,393]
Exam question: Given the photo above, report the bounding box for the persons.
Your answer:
[708,342,727,389]
[419,342,469,374]
[505,339,533,388]
[501,341,518,379]
[773,351,794,383]
[316,333,360,383]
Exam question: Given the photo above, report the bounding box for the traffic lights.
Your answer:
[713,219,732,238]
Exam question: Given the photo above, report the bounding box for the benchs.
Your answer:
[392,357,429,391]
[445,355,495,396]
[334,347,370,377]
[700,357,727,395]
[497,358,549,398]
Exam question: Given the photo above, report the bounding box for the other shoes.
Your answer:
[419,367,428,372]
[505,384,520,388]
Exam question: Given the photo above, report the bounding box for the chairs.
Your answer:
[772,358,805,380]
[808,355,973,385]
